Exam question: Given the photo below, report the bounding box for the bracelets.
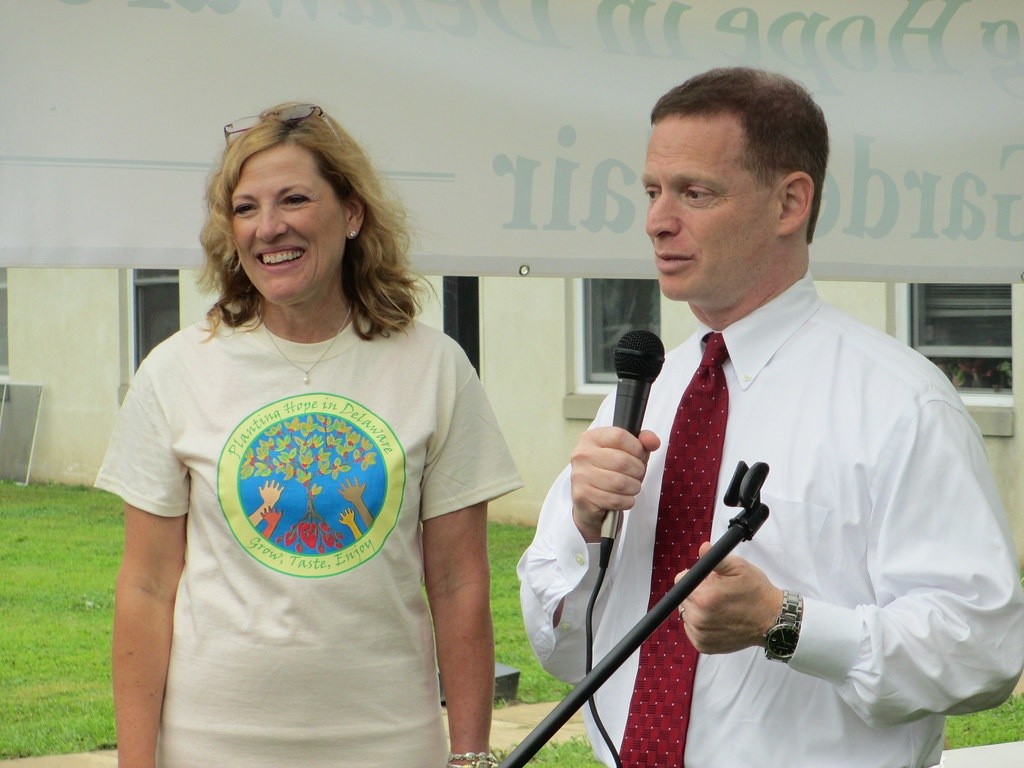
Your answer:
[445,752,494,768]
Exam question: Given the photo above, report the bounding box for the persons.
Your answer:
[514,66,1023,767]
[90,104,525,767]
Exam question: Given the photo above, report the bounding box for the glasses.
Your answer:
[223,104,340,149]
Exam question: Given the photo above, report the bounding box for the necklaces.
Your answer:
[256,297,354,386]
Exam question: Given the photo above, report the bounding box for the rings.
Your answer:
[679,607,686,620]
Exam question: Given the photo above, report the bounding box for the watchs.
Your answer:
[763,587,804,665]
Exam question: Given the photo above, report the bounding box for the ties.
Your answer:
[614,332,729,768]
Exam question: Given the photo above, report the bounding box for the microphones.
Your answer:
[598,329,665,567]
